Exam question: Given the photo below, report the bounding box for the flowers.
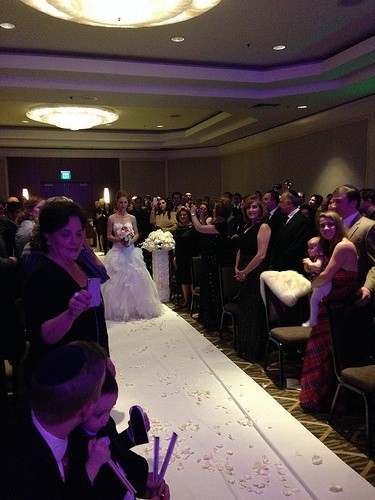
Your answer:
[140,229,175,252]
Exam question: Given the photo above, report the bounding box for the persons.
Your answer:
[0,341,170,500]
[94,183,375,410]
[300,210,357,410]
[100,191,161,322]
[302,236,332,327]
[0,195,46,396]
[22,196,115,391]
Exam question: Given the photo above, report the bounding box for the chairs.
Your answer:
[167,250,375,460]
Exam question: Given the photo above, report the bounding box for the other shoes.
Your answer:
[128,407,151,446]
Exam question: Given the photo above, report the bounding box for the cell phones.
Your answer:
[86,278,101,307]
